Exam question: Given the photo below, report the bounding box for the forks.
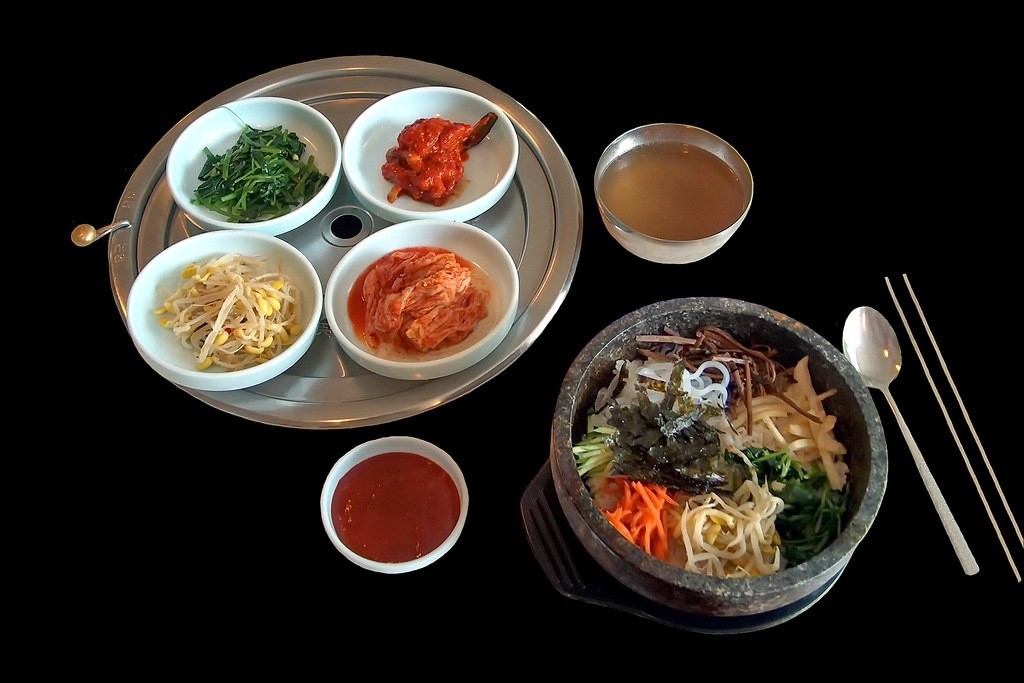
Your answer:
[839,307,983,578]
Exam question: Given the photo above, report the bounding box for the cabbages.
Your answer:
[362,249,488,352]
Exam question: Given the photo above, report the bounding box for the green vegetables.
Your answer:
[190,120,331,223]
[734,444,849,566]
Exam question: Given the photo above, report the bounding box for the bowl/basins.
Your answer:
[328,219,521,379]
[342,87,520,225]
[318,436,471,574]
[166,96,342,238]
[547,297,888,622]
[127,230,323,391]
[593,122,755,265]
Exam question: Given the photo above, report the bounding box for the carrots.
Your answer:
[595,472,681,562]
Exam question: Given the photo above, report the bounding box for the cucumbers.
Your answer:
[571,425,621,482]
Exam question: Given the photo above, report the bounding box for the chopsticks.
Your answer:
[883,272,1024,582]
